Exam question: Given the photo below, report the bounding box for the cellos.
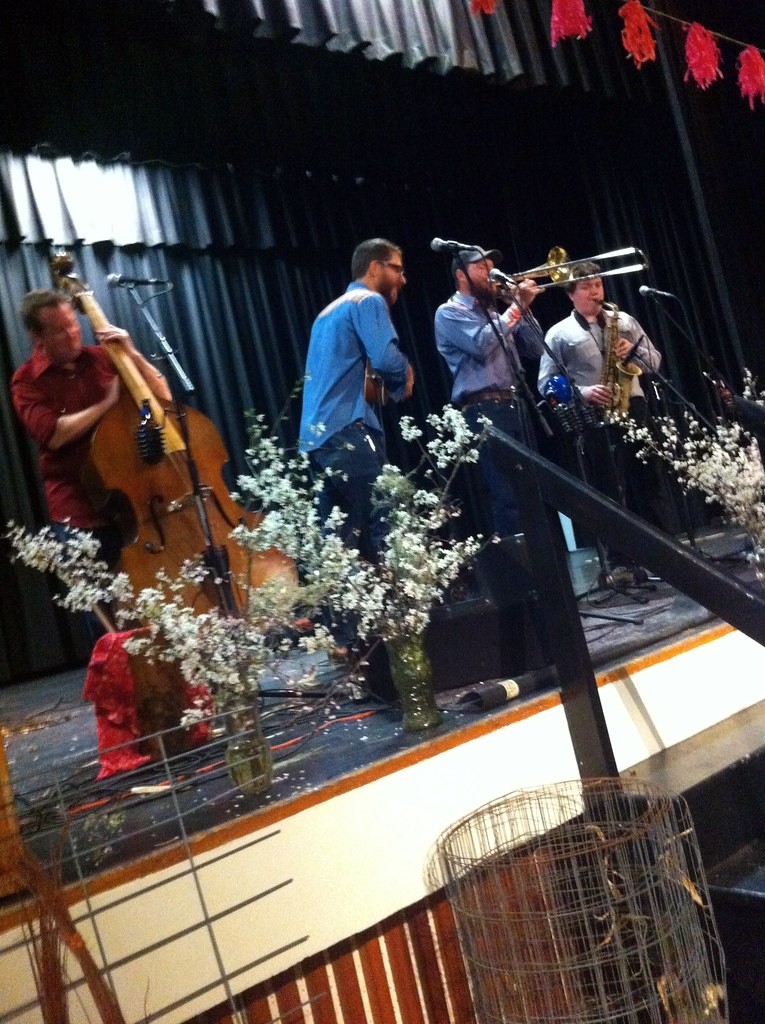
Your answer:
[51,251,298,647]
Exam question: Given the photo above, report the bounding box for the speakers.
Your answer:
[361,532,554,703]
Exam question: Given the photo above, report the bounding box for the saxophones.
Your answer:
[593,298,644,425]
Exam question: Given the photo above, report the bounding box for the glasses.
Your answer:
[376,259,404,274]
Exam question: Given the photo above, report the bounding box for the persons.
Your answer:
[11,289,171,644]
[434,252,541,543]
[536,263,662,587]
[296,239,413,704]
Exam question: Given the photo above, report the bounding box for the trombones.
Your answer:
[486,247,649,300]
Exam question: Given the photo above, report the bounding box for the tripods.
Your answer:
[455,253,672,610]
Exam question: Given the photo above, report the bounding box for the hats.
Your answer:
[452,244,502,280]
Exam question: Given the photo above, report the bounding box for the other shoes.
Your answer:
[611,566,631,586]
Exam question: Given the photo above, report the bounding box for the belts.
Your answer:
[462,390,515,408]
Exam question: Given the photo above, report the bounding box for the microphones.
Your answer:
[622,336,643,367]
[431,238,479,252]
[639,286,673,297]
[107,274,168,290]
[490,268,518,286]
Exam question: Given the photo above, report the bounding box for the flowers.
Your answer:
[702,368,765,416]
[596,410,765,588]
[8,501,348,733]
[209,397,530,644]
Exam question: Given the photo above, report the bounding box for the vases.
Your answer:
[383,627,444,732]
[216,669,274,797]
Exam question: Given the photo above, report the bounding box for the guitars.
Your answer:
[364,356,387,407]
